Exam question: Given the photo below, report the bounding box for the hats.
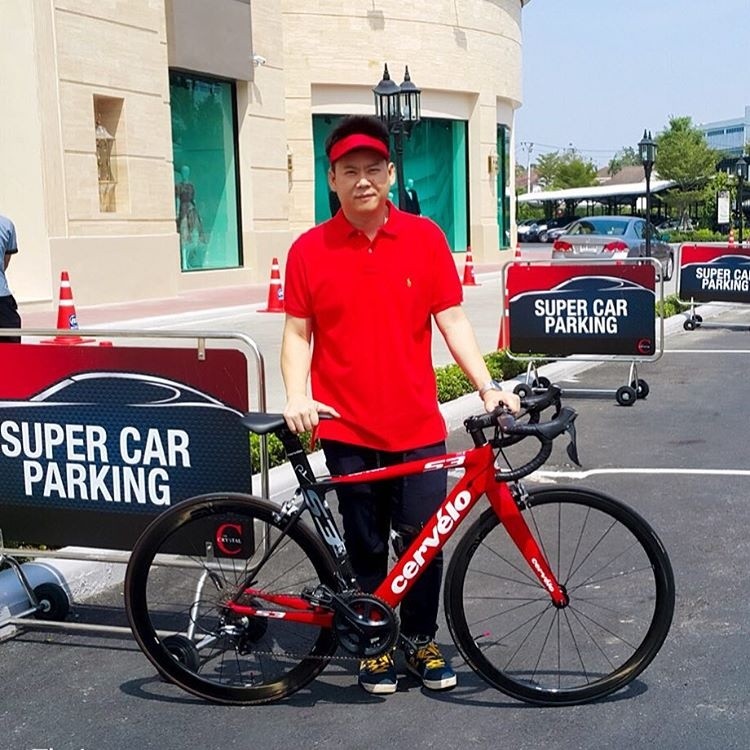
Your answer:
[330,134,388,163]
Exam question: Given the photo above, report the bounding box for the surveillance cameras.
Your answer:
[256,56,265,64]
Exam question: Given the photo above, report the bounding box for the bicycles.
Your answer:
[122,383,676,713]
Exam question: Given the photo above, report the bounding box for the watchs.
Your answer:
[478,380,502,401]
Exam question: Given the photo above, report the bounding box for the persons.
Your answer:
[177,165,202,271]
[0,215,22,343]
[405,179,422,217]
[281,115,521,694]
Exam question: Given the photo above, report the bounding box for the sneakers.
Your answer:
[359,653,398,694]
[404,634,457,690]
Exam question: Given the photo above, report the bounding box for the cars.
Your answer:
[508,216,574,244]
[550,216,675,283]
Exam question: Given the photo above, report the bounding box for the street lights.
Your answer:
[636,130,662,269]
[372,63,425,215]
[735,154,747,247]
